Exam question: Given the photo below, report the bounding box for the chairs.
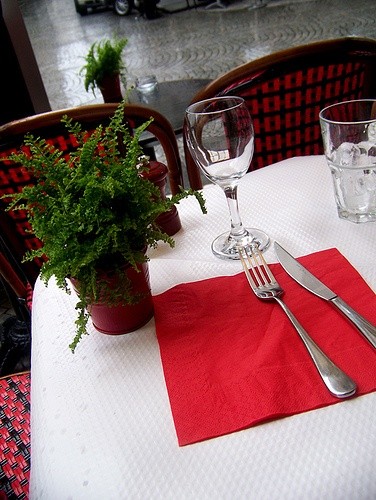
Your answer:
[182,37,376,195]
[1,103,184,500]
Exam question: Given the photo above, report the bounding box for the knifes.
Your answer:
[273,241,376,349]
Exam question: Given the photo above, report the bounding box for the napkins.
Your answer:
[152,248,375,446]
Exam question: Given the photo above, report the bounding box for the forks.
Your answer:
[237,244,357,398]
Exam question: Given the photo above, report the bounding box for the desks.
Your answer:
[123,79,213,161]
[29,156,376,500]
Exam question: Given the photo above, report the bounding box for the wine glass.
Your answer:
[183,95,271,260]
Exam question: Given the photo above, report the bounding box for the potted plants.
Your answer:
[1,100,209,353]
[79,35,128,103]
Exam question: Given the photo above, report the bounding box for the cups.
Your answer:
[319,99,376,224]
